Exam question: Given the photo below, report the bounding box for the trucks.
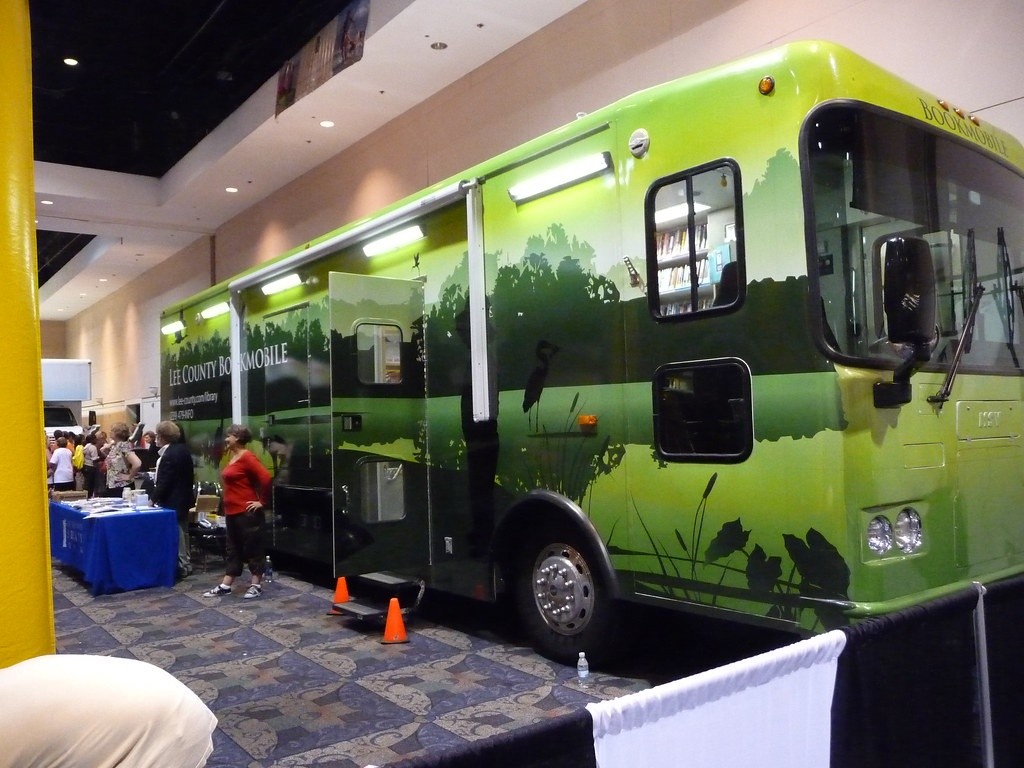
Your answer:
[40,358,91,446]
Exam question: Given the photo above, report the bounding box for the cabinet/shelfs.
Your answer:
[656,222,716,316]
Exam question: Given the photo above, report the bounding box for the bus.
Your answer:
[159,38,1024,677]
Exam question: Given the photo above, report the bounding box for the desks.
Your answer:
[48,498,179,597]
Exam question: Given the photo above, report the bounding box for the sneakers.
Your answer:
[202,585,231,597]
[243,584,263,598]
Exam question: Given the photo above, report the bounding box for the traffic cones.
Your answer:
[379,598,410,645]
[326,576,350,616]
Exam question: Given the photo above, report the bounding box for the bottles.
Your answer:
[577,652,590,689]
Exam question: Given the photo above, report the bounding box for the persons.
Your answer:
[203,424,273,599]
[142,420,194,578]
[0,653,218,768]
[44,422,142,499]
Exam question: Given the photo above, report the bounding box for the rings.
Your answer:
[252,506,254,508]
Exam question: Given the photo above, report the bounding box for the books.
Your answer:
[655,224,714,316]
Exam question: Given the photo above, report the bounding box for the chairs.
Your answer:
[192,480,226,571]
